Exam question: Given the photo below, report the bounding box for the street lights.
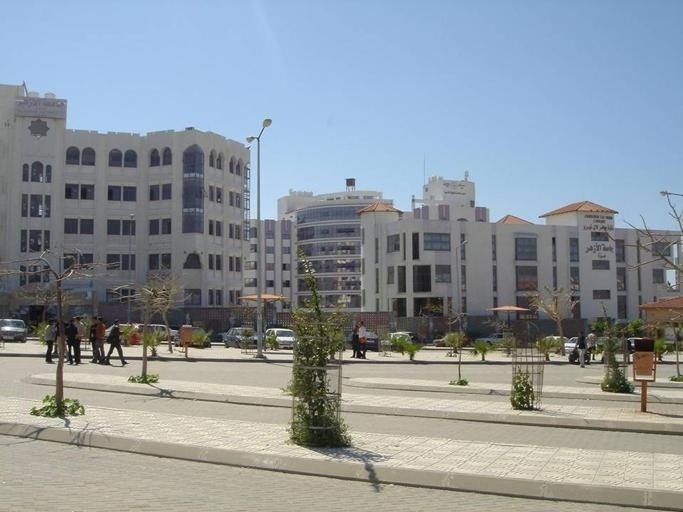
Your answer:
[246,119,272,359]
[456,240,469,345]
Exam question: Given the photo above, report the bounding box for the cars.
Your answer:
[104,324,210,348]
[0,318,27,342]
[224,327,300,350]
[345,331,381,351]
[618,338,645,353]
[535,336,597,354]
[383,331,417,351]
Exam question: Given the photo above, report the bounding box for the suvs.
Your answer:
[474,331,516,347]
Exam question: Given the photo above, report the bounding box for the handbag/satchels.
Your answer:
[360,338,367,345]
[107,334,115,344]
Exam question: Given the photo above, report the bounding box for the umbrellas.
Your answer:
[237,292,288,352]
[488,306,530,328]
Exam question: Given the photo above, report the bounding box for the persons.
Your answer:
[575,331,588,368]
[44,317,130,365]
[349,321,368,359]
[586,329,597,360]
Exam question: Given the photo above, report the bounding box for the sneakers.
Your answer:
[351,353,367,359]
[46,360,55,364]
[65,359,81,365]
[89,359,130,365]
